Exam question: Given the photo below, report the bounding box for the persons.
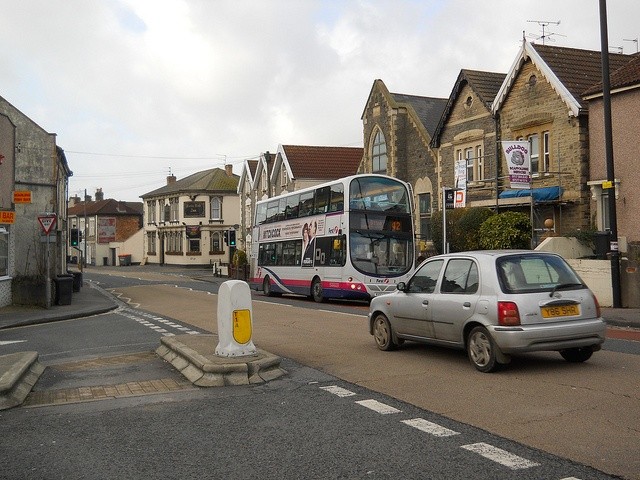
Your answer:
[302,220,317,264]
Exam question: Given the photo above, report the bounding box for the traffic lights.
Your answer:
[224,231,228,246]
[228,230,236,246]
[71,229,78,246]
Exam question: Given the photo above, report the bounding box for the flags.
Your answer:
[501,141,530,188]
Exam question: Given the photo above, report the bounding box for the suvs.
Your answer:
[367,249,606,374]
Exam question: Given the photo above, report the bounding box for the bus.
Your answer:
[251,174,416,305]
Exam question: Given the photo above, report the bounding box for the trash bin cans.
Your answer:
[67,270,81,294]
[54,275,74,305]
[58,274,73,278]
